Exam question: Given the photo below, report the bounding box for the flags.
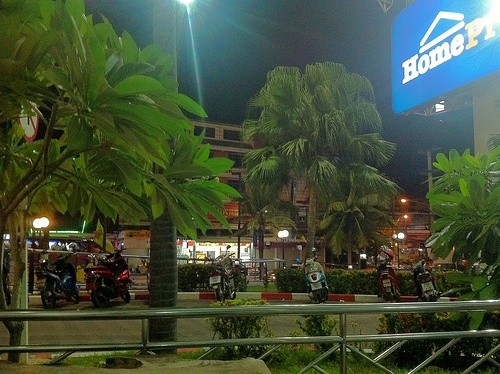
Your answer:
[93,220,115,254]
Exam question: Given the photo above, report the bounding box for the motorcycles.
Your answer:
[35,251,80,309]
[83,242,134,307]
[291,247,330,304]
[410,259,442,302]
[367,255,400,303]
[208,264,236,305]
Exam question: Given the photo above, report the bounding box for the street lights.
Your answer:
[231,170,241,258]
[278,230,289,268]
[392,233,405,270]
[32,216,50,249]
[404,214,408,250]
[400,199,434,225]
